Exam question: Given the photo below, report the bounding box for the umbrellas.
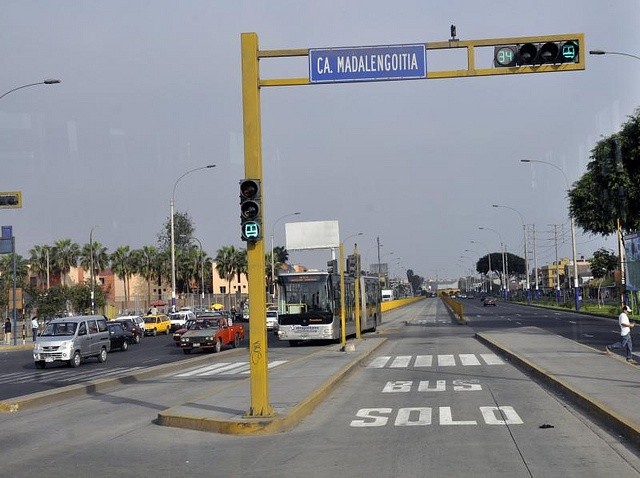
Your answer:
[150,300,168,308]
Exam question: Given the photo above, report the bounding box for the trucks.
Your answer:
[382,290,394,301]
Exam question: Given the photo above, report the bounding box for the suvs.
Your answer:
[144,314,171,336]
[118,316,144,338]
[171,311,196,330]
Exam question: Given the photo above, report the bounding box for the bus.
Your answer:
[277,272,381,346]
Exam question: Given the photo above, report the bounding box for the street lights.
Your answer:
[171,165,216,311]
[479,227,507,302]
[470,240,492,291]
[180,234,205,298]
[365,244,383,269]
[272,212,300,281]
[0,79,61,99]
[521,159,581,310]
[492,205,531,304]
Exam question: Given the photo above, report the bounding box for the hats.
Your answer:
[624,305,632,312]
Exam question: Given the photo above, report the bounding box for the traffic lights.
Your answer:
[494,40,579,67]
[327,260,337,273]
[239,179,262,241]
[0,195,17,205]
[347,255,357,275]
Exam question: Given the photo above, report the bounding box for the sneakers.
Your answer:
[627,360,637,364]
[605,345,611,354]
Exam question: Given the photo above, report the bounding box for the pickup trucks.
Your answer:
[180,317,245,354]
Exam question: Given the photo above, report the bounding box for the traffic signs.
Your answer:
[308,43,427,83]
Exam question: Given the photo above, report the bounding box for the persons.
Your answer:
[2,318,11,345]
[230,305,237,321]
[604,305,636,362]
[103,315,108,321]
[151,305,158,315]
[147,308,153,316]
[72,323,83,336]
[30,316,39,342]
[85,312,88,315]
[218,318,226,329]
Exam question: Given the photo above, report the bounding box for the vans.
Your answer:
[33,315,111,369]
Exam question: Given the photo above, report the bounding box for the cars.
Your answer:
[173,319,196,346]
[266,310,278,330]
[462,294,467,298]
[125,322,140,343]
[107,323,128,351]
[484,297,496,306]
[451,295,455,298]
